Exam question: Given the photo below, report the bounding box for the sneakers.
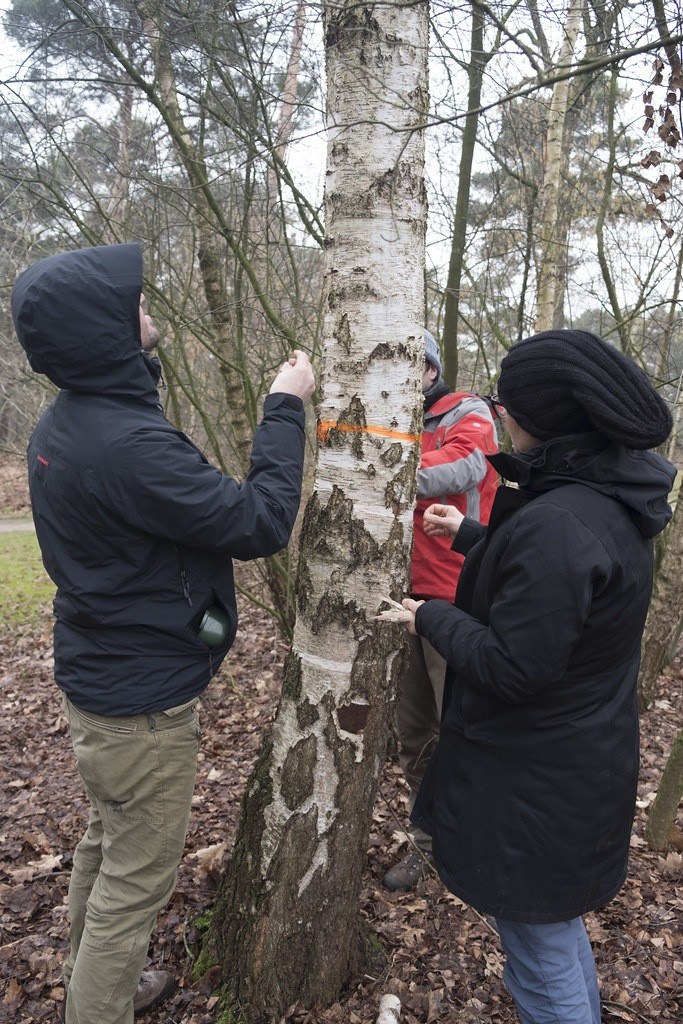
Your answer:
[134,967,177,1015]
[380,844,434,893]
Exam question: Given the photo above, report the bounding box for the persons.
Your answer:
[383,329,501,889]
[391,329,677,1024]
[9,242,320,1024]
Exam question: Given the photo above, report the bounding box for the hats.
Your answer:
[420,327,443,381]
[501,330,675,451]
